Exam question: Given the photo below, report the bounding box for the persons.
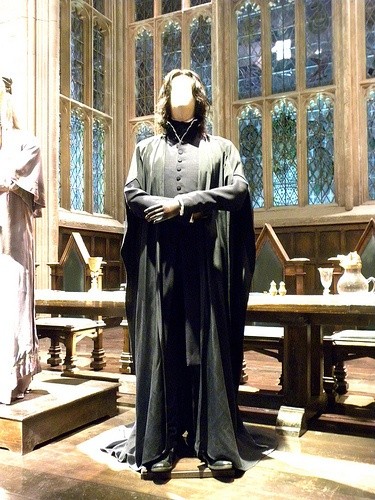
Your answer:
[0,77,46,407]
[77,69,277,474]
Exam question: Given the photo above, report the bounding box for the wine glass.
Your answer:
[88,257,102,294]
[318,268,334,295]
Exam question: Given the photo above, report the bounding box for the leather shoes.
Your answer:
[208,459,232,469]
[151,461,173,472]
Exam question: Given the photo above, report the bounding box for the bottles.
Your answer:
[278,281,286,296]
[269,280,277,296]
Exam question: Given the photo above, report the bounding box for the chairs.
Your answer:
[238,223,310,383]
[33,232,106,378]
[323,218,375,409]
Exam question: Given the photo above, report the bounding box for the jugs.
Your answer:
[337,251,375,296]
